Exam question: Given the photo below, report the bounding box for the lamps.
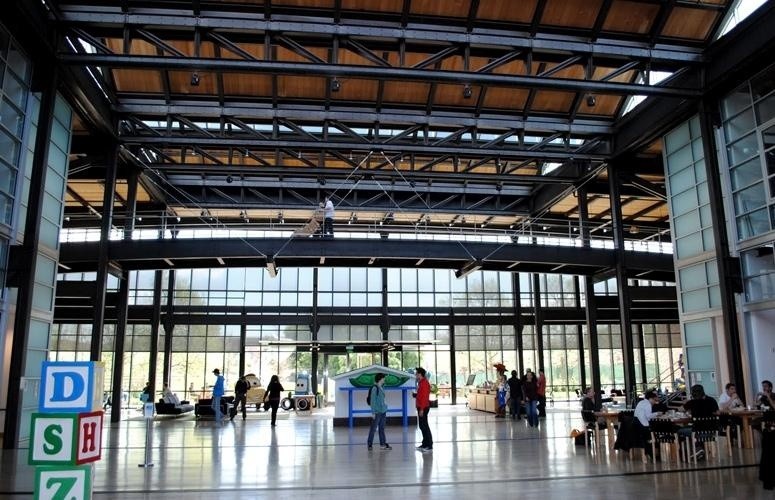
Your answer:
[184,144,508,193]
[187,67,606,109]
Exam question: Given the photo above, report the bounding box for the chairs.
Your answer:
[574,384,627,402]
[582,400,765,471]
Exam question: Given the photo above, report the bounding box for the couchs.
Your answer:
[153,391,237,422]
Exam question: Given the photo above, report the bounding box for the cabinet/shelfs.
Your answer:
[468,386,510,414]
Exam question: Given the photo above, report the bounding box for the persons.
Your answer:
[582,380,775,491]
[138,381,209,410]
[322,197,336,239]
[315,201,325,239]
[678,354,686,379]
[263,375,284,427]
[210,368,231,428]
[408,368,434,450]
[230,376,250,420]
[495,368,546,428]
[366,372,392,452]
